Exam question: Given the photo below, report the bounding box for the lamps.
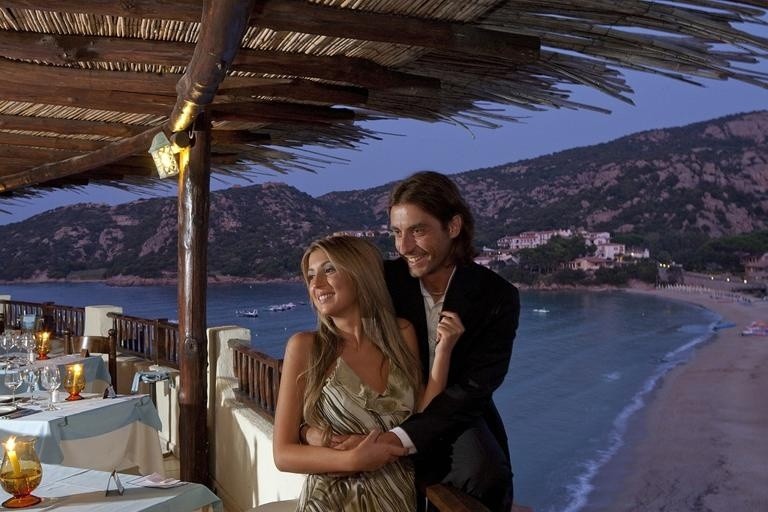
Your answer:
[148,131,180,180]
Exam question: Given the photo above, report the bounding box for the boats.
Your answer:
[533,309,552,314]
[237,302,296,316]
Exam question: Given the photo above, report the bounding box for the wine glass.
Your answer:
[62,362,89,402]
[0,326,64,416]
[1,434,43,509]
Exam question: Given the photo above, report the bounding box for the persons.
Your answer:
[298,169,522,511]
[271,228,467,511]
